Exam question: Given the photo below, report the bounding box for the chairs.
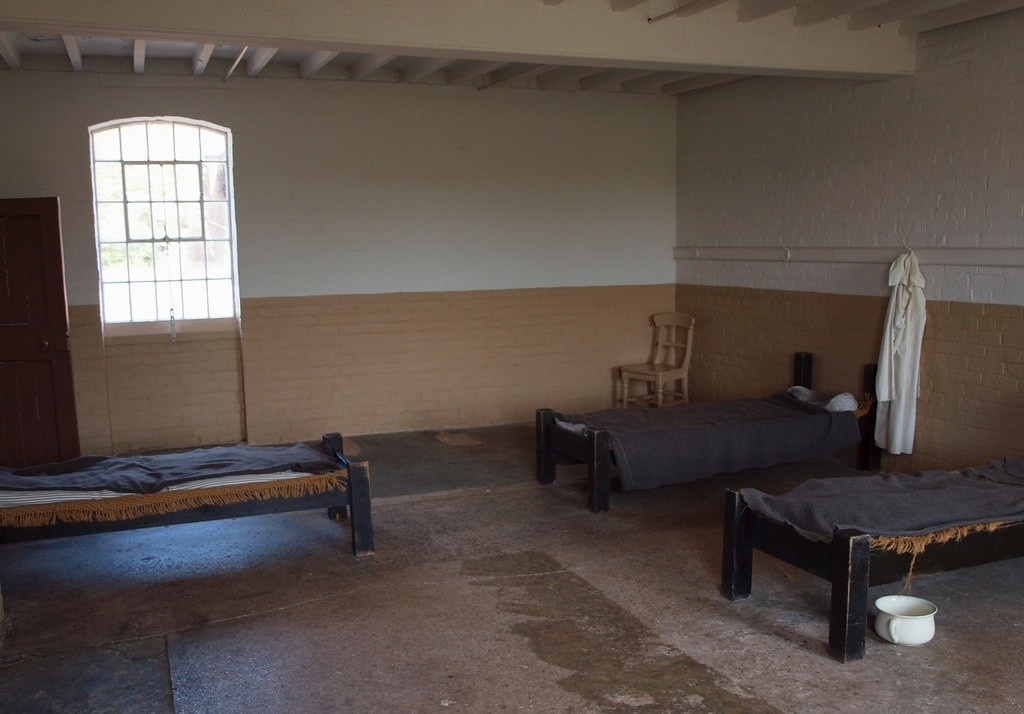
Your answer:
[619,312,695,408]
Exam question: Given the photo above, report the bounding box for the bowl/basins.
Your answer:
[874,594,938,648]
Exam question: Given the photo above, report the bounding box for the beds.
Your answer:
[535,352,882,515]
[0,431,376,557]
[721,455,1024,665]
